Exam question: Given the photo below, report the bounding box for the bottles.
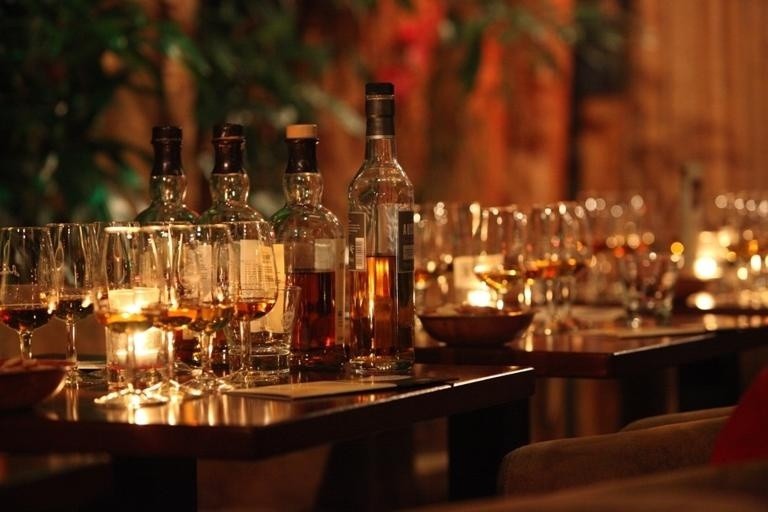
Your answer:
[132,125,201,223]
[265,124,346,370]
[195,123,277,242]
[349,78,415,371]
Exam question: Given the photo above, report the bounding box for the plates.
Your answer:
[416,308,537,345]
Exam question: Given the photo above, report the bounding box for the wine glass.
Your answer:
[93,220,140,240]
[94,236,167,405]
[0,226,58,360]
[415,201,453,307]
[177,225,241,392]
[46,221,100,384]
[216,220,279,384]
[475,201,594,332]
[139,225,202,401]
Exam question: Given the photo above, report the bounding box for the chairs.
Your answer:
[423,366,767,511]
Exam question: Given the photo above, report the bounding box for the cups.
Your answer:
[618,254,677,328]
[225,285,303,375]
